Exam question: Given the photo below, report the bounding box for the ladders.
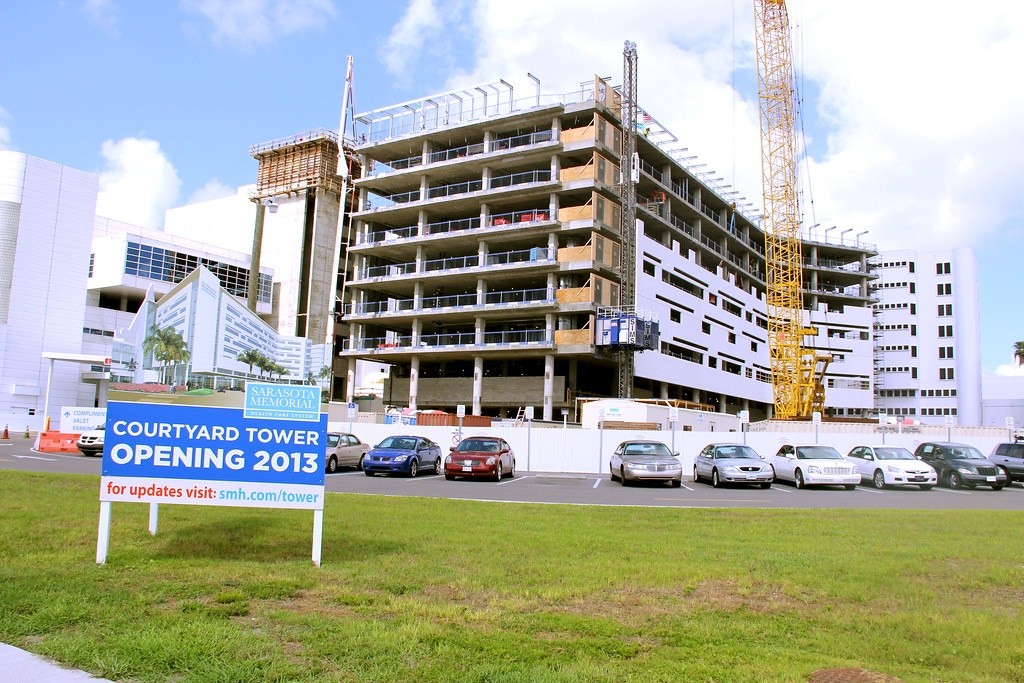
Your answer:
[513,407,526,427]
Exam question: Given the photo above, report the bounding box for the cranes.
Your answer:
[748,0,837,419]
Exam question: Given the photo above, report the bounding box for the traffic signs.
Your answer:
[59,406,107,435]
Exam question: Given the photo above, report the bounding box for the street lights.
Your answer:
[245,195,279,314]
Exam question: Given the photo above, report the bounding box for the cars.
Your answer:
[844,443,938,491]
[362,435,443,478]
[75,421,106,457]
[765,441,862,490]
[325,430,371,474]
[609,440,682,488]
[443,437,516,482]
[693,441,774,489]
[913,440,1007,492]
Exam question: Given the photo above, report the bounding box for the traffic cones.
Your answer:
[45,417,51,430]
[22,425,31,439]
[1,423,10,440]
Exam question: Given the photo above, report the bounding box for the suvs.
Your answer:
[987,437,1024,487]
